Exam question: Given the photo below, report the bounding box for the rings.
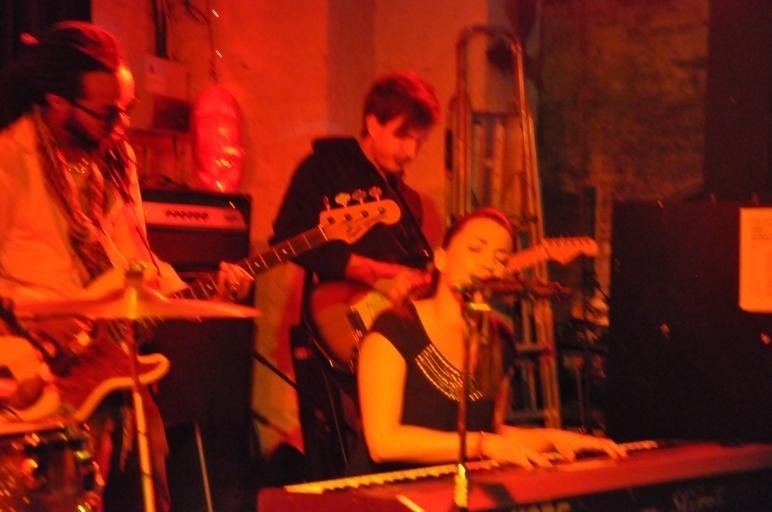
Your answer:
[230,285,241,301]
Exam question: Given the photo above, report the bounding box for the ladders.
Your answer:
[455,20,562,431]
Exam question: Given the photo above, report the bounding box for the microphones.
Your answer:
[120,261,145,349]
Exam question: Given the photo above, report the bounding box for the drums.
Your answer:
[0,416,105,512]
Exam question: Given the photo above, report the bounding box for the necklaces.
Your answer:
[55,149,89,176]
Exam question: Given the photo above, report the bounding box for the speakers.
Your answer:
[609,199,772,512]
[139,271,252,512]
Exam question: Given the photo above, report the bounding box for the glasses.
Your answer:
[61,95,125,124]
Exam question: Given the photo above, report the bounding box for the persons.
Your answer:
[0,22,261,384]
[142,0,257,134]
[345,208,628,472]
[265,67,440,475]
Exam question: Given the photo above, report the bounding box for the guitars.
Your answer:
[0,186,401,423]
[301,233,599,377]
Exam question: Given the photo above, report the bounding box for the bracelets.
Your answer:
[473,428,488,460]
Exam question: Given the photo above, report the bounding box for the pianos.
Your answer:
[256,439,772,512]
